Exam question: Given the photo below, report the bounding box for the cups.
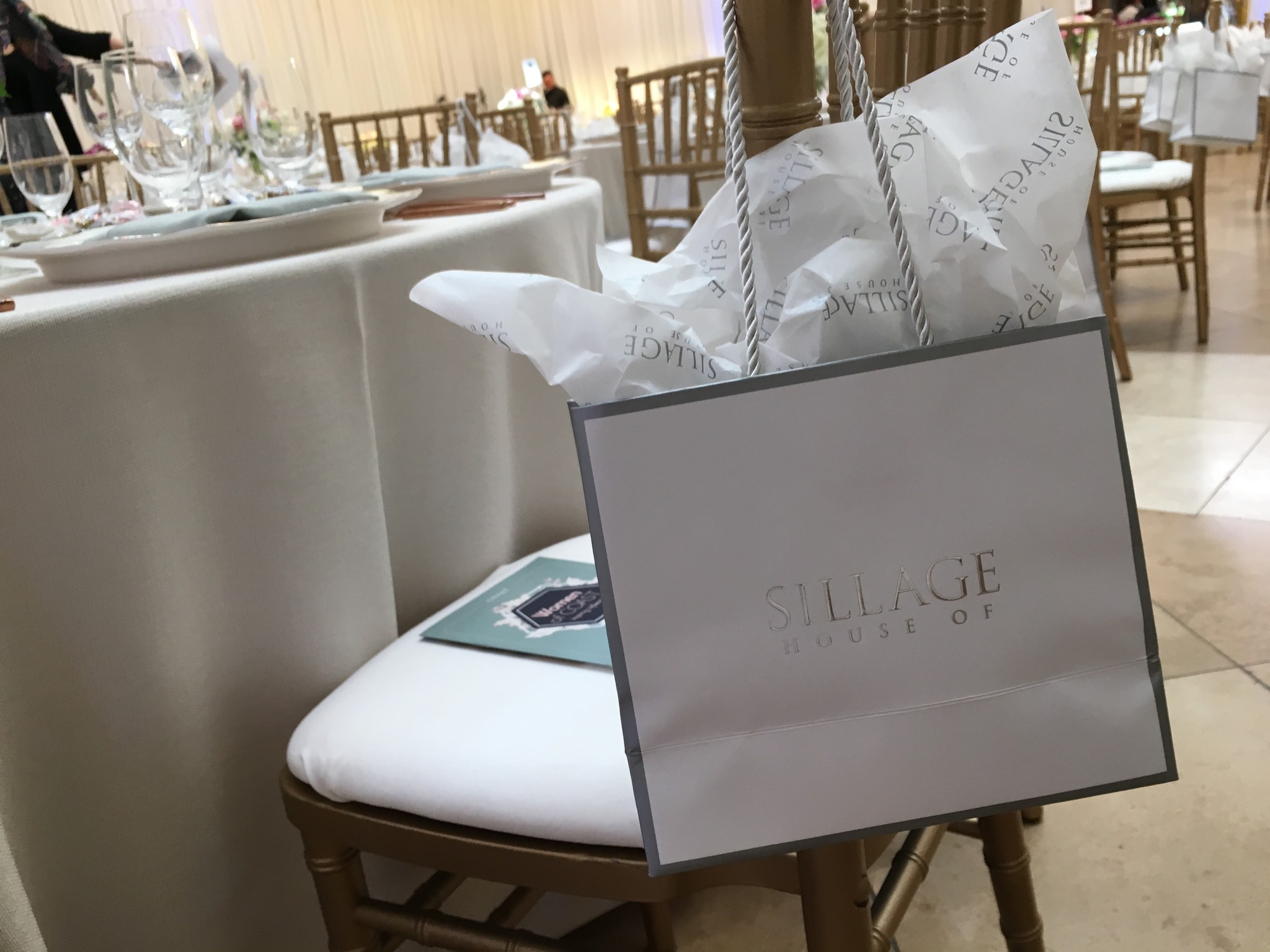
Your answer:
[184,117,230,210]
[122,5,216,135]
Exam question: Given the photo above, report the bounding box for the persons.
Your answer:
[1112,0,1161,22]
[542,72,570,109]
[0,0,181,216]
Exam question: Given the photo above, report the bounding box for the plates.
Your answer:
[361,156,570,215]
[0,187,423,285]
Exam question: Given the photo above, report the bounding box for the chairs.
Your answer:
[0,1,1270,952]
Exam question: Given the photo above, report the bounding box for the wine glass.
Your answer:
[240,53,323,195]
[1,110,85,242]
[74,62,144,208]
[101,43,198,216]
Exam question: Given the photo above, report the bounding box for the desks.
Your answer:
[570,133,725,250]
[0,174,605,951]
